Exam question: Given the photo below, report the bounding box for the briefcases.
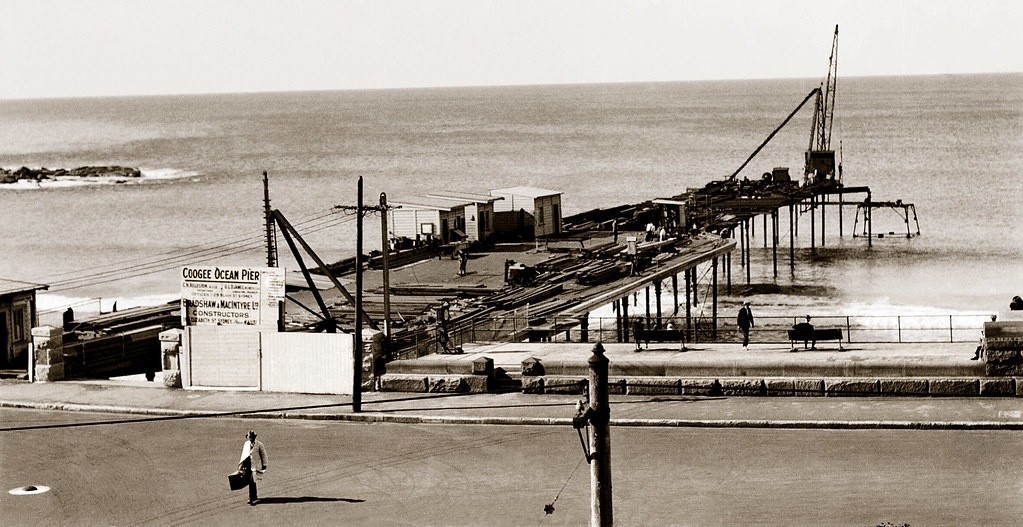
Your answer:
[228,470,248,490]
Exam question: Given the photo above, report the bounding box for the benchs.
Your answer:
[632,329,688,352]
[788,329,843,352]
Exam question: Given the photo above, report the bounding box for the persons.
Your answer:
[737,301,754,348]
[612,216,618,244]
[437,324,449,354]
[804,315,817,350]
[457,248,467,275]
[971,296,1023,360]
[838,162,842,180]
[744,176,750,186]
[633,317,676,348]
[238,430,268,504]
[645,217,697,253]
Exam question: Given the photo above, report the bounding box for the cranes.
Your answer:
[806,22,841,183]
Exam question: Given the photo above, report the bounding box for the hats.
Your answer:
[246,430,257,440]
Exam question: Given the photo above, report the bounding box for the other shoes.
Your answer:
[247,499,258,504]
